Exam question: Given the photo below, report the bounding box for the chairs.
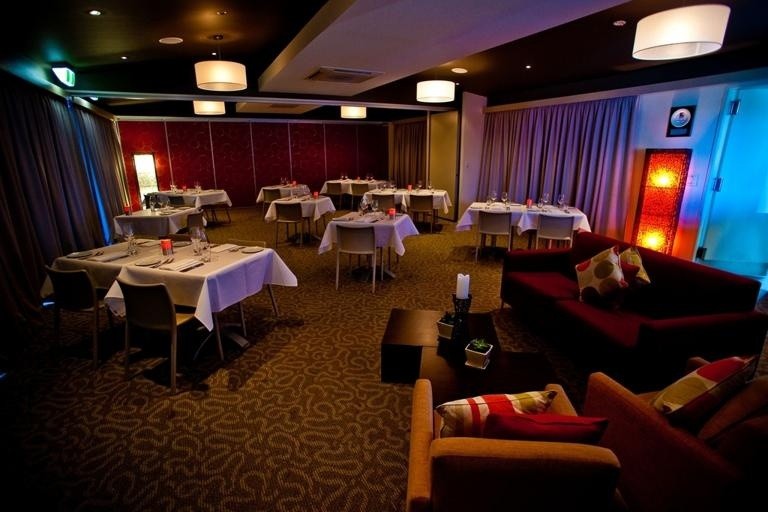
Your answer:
[581,354,768,512]
[406,379,626,512]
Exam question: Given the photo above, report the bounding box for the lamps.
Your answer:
[416,68,455,103]
[194,34,248,92]
[631,148,692,256]
[51,62,78,88]
[193,101,226,115]
[632,0,732,61]
[340,106,367,119]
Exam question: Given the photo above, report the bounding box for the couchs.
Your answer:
[500,231,767,394]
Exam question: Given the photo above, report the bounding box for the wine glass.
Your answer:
[340,174,374,181]
[148,194,170,216]
[415,180,435,196]
[170,182,202,193]
[281,177,290,186]
[291,190,313,201]
[360,196,379,219]
[190,227,210,262]
[120,222,136,255]
[483,191,571,214]
[377,179,397,192]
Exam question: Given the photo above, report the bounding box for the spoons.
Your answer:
[80,250,104,259]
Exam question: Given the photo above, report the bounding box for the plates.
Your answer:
[134,258,160,266]
[173,240,190,248]
[241,247,265,253]
[66,251,92,257]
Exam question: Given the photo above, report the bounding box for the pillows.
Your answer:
[435,389,558,438]
[620,245,651,292]
[651,354,758,425]
[689,378,768,442]
[483,412,610,445]
[574,245,628,303]
